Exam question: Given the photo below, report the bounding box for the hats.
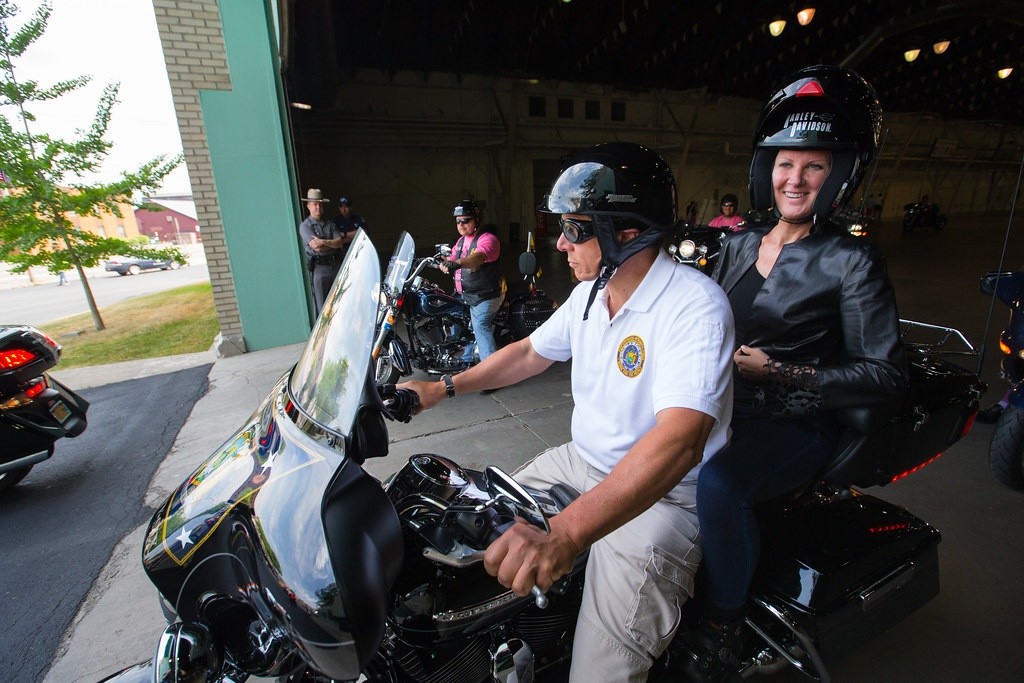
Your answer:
[337,196,352,205]
[300,188,330,203]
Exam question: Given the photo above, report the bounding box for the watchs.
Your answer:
[439,374,456,398]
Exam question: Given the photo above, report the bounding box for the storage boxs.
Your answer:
[0,326,62,386]
[515,299,557,337]
[845,321,986,485]
[767,489,945,652]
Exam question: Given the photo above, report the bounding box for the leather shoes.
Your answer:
[673,615,746,683]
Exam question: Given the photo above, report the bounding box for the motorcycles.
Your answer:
[362,228,562,391]
[658,200,766,276]
[96,229,989,683]
[973,262,1024,492]
[0,324,89,497]
[903,194,951,234]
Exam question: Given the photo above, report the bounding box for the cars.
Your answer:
[104,248,181,274]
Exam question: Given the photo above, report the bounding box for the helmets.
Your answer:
[536,141,682,231]
[720,193,739,215]
[748,62,884,225]
[453,199,481,223]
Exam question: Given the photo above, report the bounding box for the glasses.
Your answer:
[558,217,597,244]
[455,217,473,225]
[724,203,734,207]
[340,205,349,207]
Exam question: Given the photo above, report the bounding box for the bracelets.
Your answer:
[343,231,347,238]
[458,259,462,267]
[327,239,330,246]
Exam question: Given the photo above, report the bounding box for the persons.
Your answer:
[975,386,1013,424]
[397,142,733,683]
[707,194,748,231]
[298,189,344,319]
[333,196,370,258]
[860,193,934,223]
[428,199,507,395]
[672,62,906,683]
